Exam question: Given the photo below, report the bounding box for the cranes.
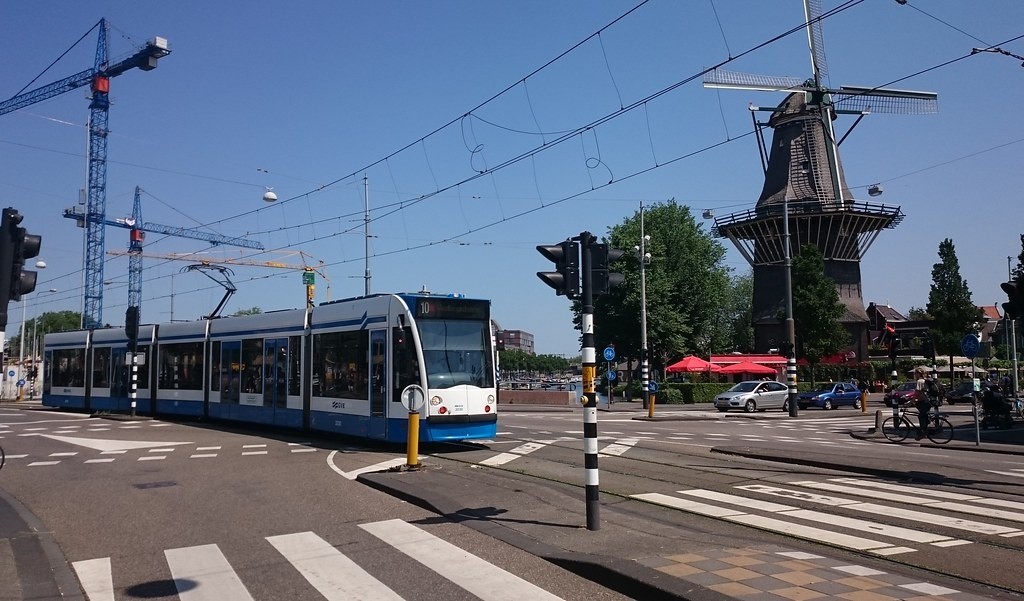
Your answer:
[103,248,331,309]
[0,16,173,332]
[61,184,266,327]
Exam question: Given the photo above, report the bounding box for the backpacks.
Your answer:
[921,378,944,406]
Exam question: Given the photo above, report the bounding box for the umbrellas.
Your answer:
[908,365,938,377]
[939,366,965,378]
[719,360,778,380]
[963,365,987,378]
[667,355,722,383]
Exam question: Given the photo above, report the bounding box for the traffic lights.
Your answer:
[1000,277,1024,321]
[10,227,43,303]
[536,240,580,297]
[987,331,1000,348]
[592,244,627,295]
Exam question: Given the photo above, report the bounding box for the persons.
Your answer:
[909,367,934,441]
[1001,372,1016,398]
[838,389,844,395]
[851,378,871,393]
[991,386,1015,423]
[986,373,989,381]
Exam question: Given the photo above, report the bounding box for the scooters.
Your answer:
[972,386,1024,431]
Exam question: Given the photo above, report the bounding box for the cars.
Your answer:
[945,379,994,406]
[713,380,791,413]
[797,381,862,410]
[883,381,921,408]
[499,374,583,390]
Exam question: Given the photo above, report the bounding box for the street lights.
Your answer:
[29,288,57,401]
[629,200,652,410]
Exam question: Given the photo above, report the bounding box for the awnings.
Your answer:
[710,357,787,364]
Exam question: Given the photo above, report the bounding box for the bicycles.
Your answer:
[882,395,954,444]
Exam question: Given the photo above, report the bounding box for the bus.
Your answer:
[41,285,500,449]
